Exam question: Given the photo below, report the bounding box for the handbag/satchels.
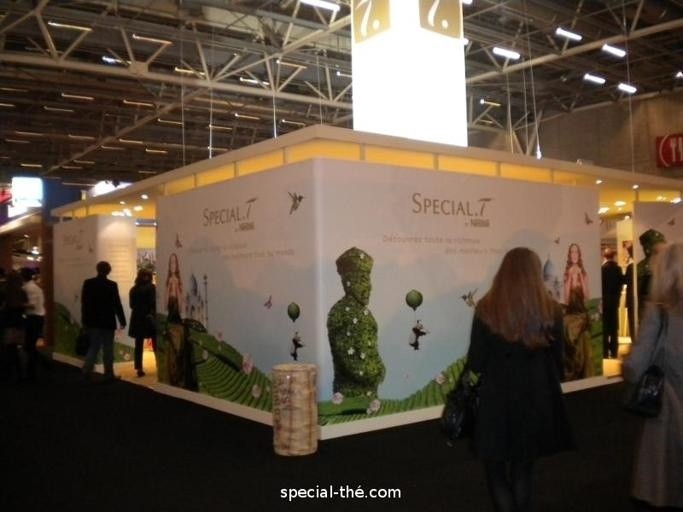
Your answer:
[442,380,474,447]
[619,365,664,418]
[75,337,92,354]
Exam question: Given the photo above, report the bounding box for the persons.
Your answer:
[128,267,157,378]
[465,248,582,512]
[622,243,682,511]
[0,267,57,392]
[79,262,126,389]
[560,245,589,382]
[622,246,634,344]
[165,253,185,365]
[602,249,623,358]
